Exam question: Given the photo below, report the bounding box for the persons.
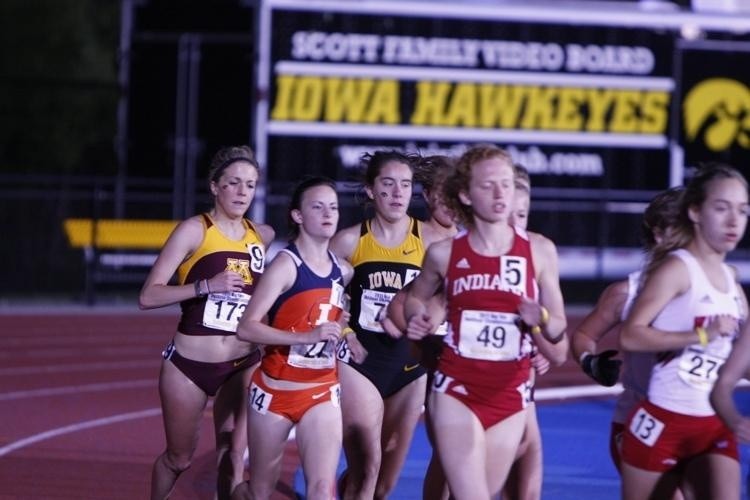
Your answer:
[232,175,355,499]
[131,142,264,500]
[323,142,572,500]
[615,162,744,499]
[706,316,750,446]
[571,184,712,500]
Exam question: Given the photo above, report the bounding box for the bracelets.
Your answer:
[205,279,211,294]
[194,280,204,298]
[695,326,710,348]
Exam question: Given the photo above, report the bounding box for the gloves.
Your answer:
[580,349,622,387]
